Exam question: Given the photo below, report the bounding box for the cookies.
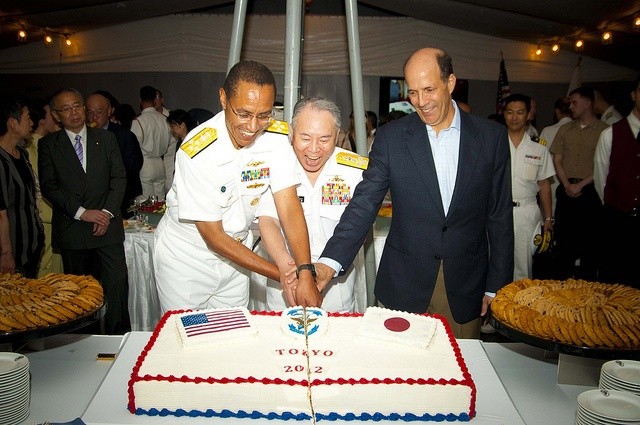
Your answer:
[0,272,104,334]
[489,277,639,348]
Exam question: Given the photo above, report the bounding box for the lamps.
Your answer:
[552,41,560,56]
[633,14,639,28]
[602,23,613,44]
[43,31,53,46]
[533,42,543,58]
[18,25,27,41]
[64,33,72,47]
[575,30,584,48]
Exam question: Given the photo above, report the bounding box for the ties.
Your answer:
[74,135,83,167]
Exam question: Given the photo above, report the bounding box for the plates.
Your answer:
[0,352,31,424]
[573,358,640,425]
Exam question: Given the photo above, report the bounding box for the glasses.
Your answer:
[229,98,276,122]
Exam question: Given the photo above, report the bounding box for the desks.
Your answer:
[121,210,162,332]
[480,341,606,425]
[0,331,525,425]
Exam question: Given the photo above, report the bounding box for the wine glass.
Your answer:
[136,209,145,235]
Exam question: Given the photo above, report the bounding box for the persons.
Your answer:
[149,59,289,320]
[539,97,576,218]
[26,97,61,279]
[164,108,196,179]
[284,46,515,339]
[128,85,170,201]
[257,95,369,308]
[86,93,144,220]
[36,85,132,336]
[525,95,541,142]
[488,113,507,126]
[365,111,377,155]
[549,87,609,234]
[1,97,45,280]
[592,89,623,126]
[342,111,374,153]
[500,93,555,283]
[592,83,639,290]
[456,99,471,114]
[152,89,177,190]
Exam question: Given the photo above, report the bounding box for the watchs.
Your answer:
[295,264,317,280]
[545,216,556,225]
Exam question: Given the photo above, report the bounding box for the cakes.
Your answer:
[127,306,477,422]
